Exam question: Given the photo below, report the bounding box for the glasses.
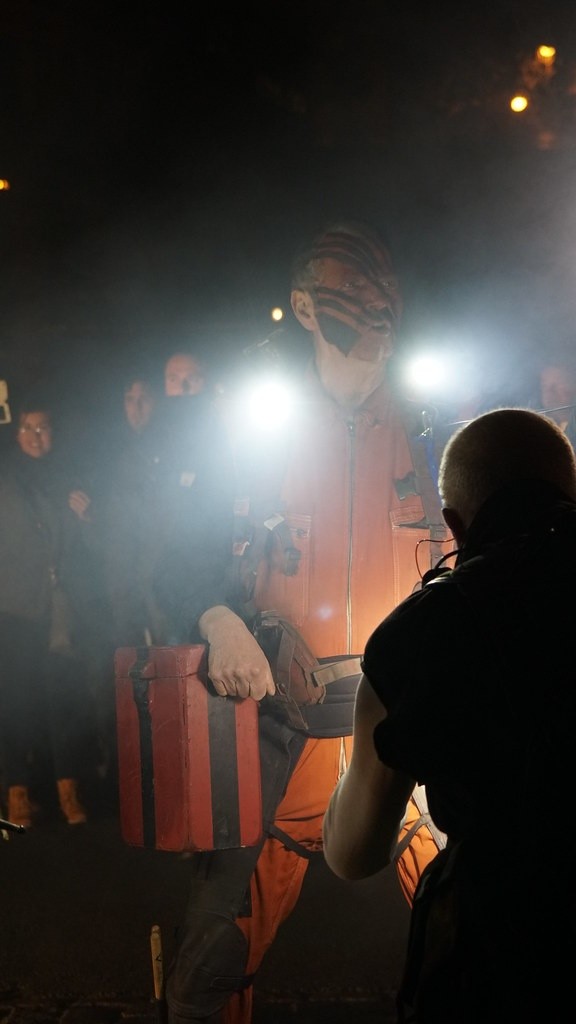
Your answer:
[18,422,52,433]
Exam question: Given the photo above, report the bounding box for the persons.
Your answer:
[0,342,211,851]
[147,215,448,1024]
[320,408,576,1023]
[530,355,576,453]
